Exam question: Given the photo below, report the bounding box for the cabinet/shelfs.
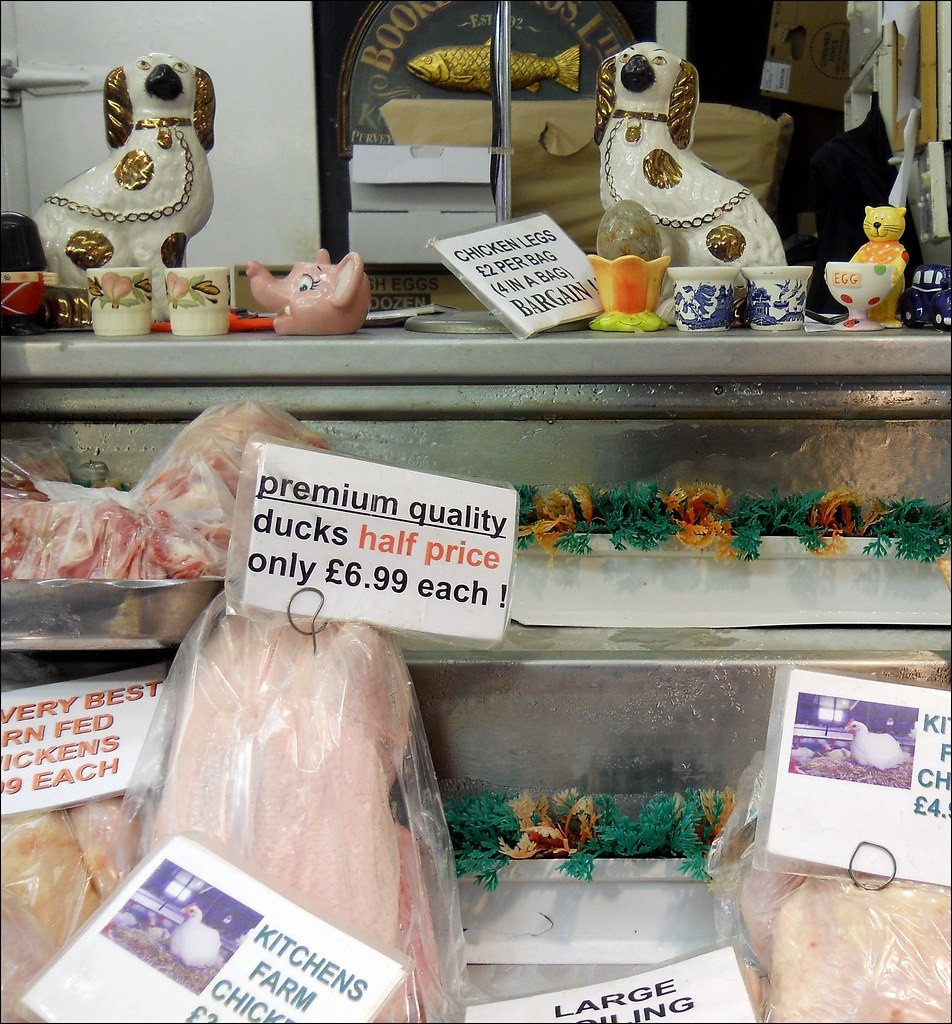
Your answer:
[2,332,951,1023]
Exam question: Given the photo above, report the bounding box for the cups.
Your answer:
[164,265,231,337]
[667,265,740,331]
[1,270,48,337]
[86,267,153,335]
[587,254,672,331]
[740,265,813,331]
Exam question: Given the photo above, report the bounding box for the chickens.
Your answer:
[169,902,221,969]
[844,717,904,770]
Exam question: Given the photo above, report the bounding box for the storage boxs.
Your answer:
[457,853,725,969]
[343,143,499,273]
[513,527,951,632]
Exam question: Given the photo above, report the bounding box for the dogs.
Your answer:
[31,52,216,322]
[594,42,789,326]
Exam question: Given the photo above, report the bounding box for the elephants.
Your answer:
[245,247,371,336]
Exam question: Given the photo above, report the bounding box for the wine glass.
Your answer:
[825,261,895,331]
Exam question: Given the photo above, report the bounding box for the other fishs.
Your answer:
[408,38,580,95]
